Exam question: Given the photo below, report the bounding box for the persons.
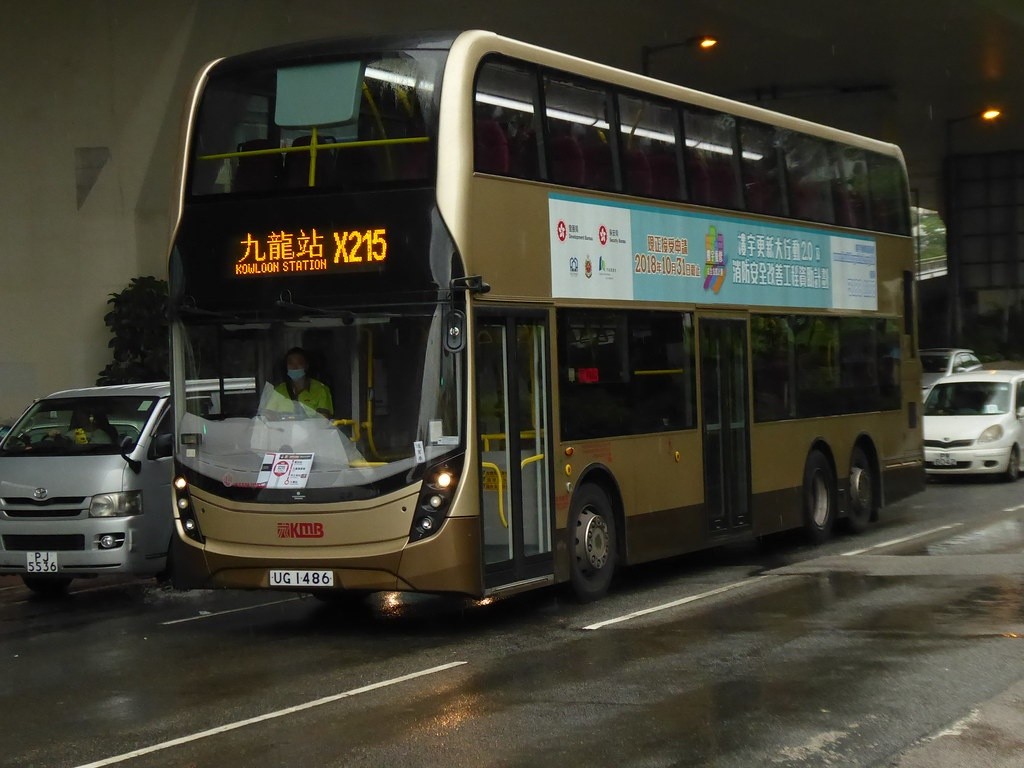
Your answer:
[255,347,334,422]
[47,410,117,445]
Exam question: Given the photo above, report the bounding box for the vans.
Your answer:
[0,377,257,598]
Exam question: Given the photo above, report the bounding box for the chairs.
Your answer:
[953,391,986,410]
[93,414,118,443]
[753,336,894,421]
[236,111,897,234]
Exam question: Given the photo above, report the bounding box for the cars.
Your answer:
[922,369,1024,484]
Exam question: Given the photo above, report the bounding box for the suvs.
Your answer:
[918,348,985,405]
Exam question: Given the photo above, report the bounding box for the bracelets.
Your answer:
[281,415,286,420]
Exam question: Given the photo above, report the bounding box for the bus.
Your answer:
[161,28,928,614]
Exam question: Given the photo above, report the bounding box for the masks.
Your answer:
[287,368,305,379]
